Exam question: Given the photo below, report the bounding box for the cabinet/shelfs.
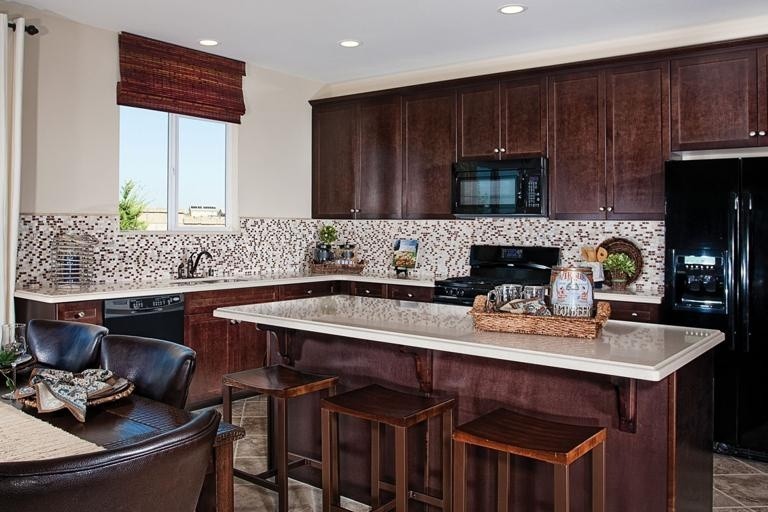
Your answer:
[279,281,331,301]
[672,42,768,151]
[332,280,433,303]
[456,75,547,160]
[312,95,403,220]
[184,286,278,412]
[404,88,457,220]
[593,299,662,324]
[56,300,103,325]
[548,60,671,220]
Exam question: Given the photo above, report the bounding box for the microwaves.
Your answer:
[450,155,547,217]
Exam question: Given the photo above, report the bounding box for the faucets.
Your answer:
[187,251,212,278]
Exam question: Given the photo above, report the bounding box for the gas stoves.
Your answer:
[433,276,550,307]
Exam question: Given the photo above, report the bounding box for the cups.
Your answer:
[495,284,522,304]
[524,285,545,303]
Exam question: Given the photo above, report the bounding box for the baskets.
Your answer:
[311,259,368,273]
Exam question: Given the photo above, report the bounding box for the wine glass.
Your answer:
[0,323,32,402]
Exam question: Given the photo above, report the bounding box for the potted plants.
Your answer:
[601,253,636,291]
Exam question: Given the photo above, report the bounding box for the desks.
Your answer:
[213,295,725,512]
[0,361,246,512]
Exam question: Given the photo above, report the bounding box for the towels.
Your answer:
[2,366,127,423]
[467,295,611,339]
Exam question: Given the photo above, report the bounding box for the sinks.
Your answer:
[202,279,248,283]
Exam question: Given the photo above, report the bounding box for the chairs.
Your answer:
[101,335,197,409]
[0,408,222,512]
[27,319,109,373]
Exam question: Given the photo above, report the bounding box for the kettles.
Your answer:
[334,244,356,265]
[314,242,334,264]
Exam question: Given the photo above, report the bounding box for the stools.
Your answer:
[320,383,455,512]
[222,364,339,511]
[452,407,607,512]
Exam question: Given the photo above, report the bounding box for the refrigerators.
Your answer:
[665,146,768,462]
